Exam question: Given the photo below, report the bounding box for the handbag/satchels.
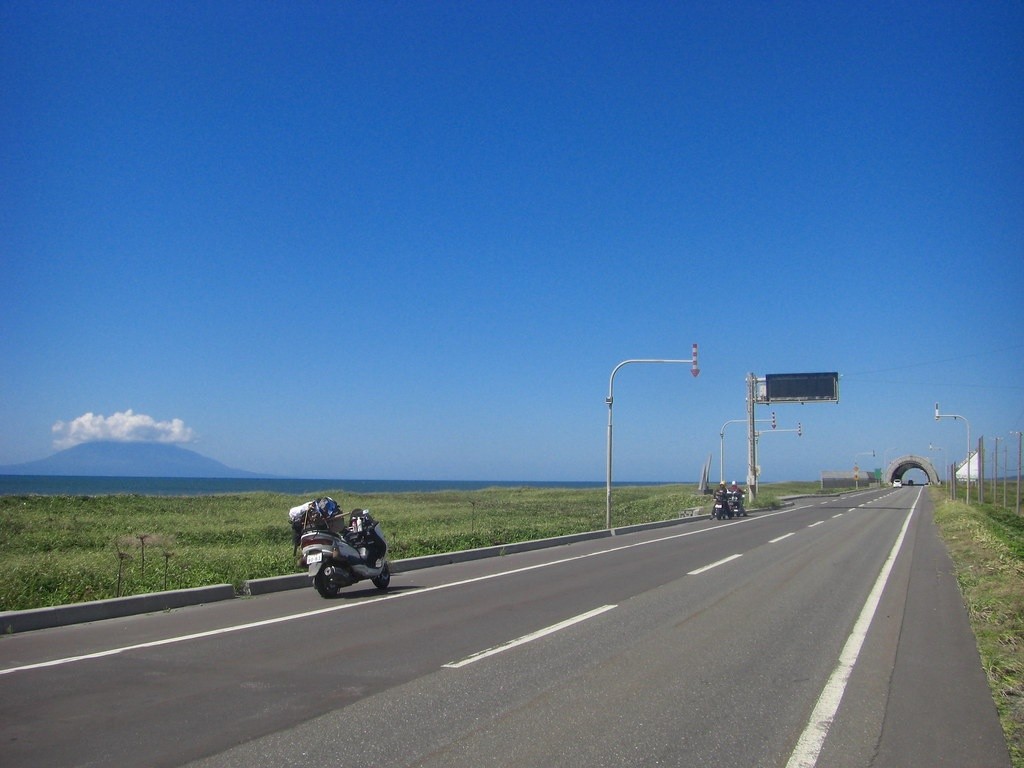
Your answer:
[288,496,340,528]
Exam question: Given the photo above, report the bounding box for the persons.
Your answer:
[710,481,732,520]
[730,481,748,516]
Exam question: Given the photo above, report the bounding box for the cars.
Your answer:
[908,480,913,486]
[893,479,902,488]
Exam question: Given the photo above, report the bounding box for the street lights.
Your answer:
[719,411,776,482]
[935,402,970,506]
[604,343,700,530]
[753,423,802,493]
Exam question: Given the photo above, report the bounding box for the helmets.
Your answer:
[720,481,726,486]
[731,480,737,487]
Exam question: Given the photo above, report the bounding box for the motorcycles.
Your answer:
[713,488,747,520]
[287,496,391,598]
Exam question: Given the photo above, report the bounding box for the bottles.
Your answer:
[352,517,357,532]
[356,517,363,532]
